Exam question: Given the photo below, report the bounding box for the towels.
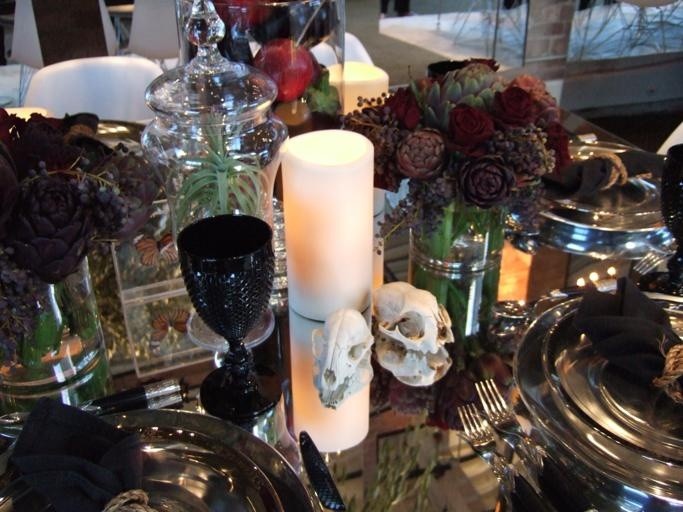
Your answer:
[573,276,683,398]
[0,396,144,512]
[540,142,667,202]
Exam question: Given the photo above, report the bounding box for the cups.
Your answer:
[404,186,508,273]
[406,254,499,349]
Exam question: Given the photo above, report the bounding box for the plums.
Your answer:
[214,0,337,65]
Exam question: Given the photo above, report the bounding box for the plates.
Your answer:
[1,423,285,512]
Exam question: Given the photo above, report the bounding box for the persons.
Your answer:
[379,0,415,20]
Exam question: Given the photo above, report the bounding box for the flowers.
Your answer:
[345,57,581,241]
[370,338,516,431]
[0,111,170,368]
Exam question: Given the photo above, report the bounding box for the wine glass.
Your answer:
[176,213,282,424]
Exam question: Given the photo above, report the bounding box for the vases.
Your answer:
[409,198,505,271]
[409,259,500,332]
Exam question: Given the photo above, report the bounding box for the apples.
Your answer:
[252,38,314,102]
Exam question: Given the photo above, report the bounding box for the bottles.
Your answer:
[142,1,290,352]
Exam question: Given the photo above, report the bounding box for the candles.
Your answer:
[279,129,374,322]
[287,308,371,453]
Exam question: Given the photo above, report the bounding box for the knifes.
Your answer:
[298,430,347,511]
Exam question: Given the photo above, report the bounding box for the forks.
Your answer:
[456,379,597,511]
[632,247,672,276]
